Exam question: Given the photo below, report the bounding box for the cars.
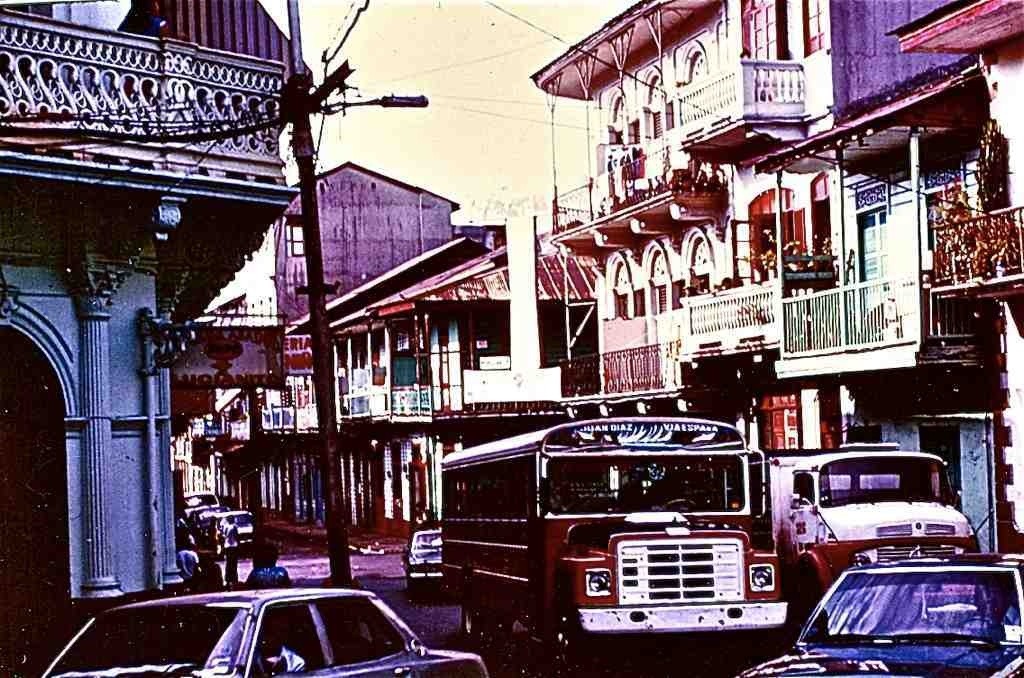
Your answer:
[181,490,252,554]
[36,585,486,678]
[737,558,1021,678]
[770,440,982,636]
[400,527,442,589]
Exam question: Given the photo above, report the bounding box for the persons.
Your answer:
[223,516,241,588]
[249,611,308,678]
[174,511,204,579]
[245,541,291,588]
[618,482,690,512]
[963,584,1021,642]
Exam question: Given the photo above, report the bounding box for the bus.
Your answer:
[441,412,788,644]
